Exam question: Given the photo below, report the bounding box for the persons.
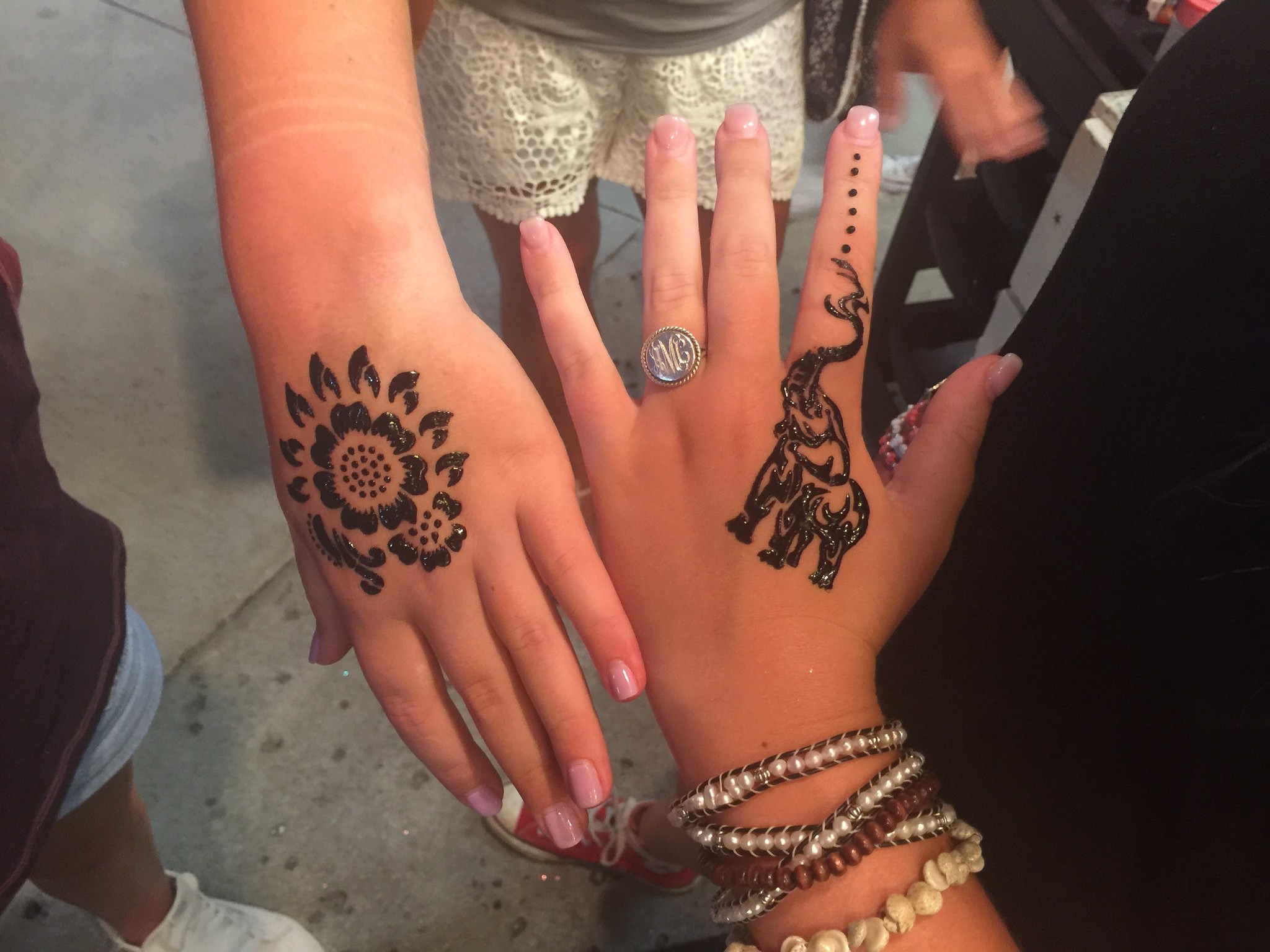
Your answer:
[182,0,1270,952]
[0,240,324,952]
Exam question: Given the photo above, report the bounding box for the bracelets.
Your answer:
[683,800,955,857]
[711,750,926,925]
[723,823,984,952]
[696,771,941,888]
[666,724,956,825]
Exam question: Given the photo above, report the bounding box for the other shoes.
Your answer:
[101,867,323,952]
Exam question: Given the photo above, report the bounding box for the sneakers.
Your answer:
[484,781,704,893]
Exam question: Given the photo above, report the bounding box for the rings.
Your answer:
[639,327,705,388]
